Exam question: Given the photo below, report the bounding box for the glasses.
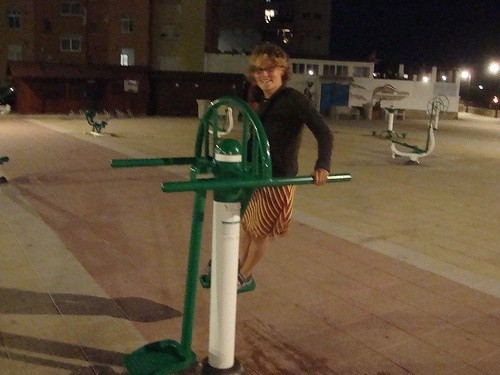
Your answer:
[251,64,279,74]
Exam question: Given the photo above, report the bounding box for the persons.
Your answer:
[208,40,334,289]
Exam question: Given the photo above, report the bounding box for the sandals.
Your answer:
[237,273,253,290]
